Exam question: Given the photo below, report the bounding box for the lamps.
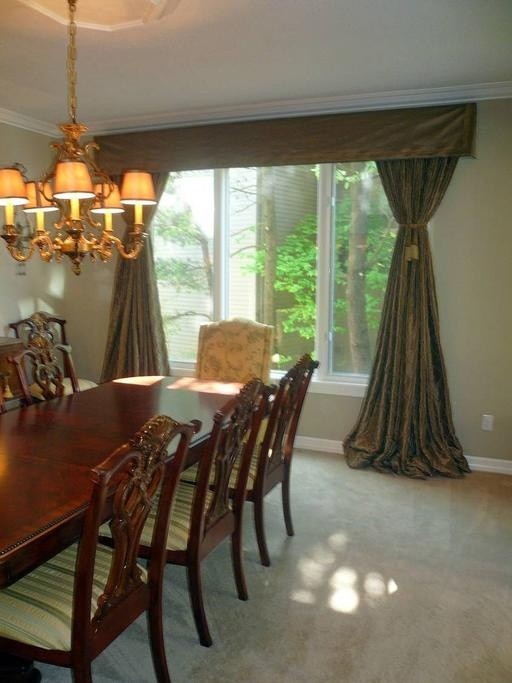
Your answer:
[1,0,160,277]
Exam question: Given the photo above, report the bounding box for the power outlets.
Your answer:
[481,414,495,431]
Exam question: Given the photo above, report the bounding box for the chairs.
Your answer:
[180,352,320,567]
[8,344,80,407]
[196,319,276,386]
[96,376,277,648]
[9,310,99,401]
[0,413,203,683]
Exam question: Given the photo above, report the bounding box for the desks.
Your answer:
[0,371,287,595]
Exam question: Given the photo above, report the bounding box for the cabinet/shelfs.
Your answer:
[0,337,24,415]
